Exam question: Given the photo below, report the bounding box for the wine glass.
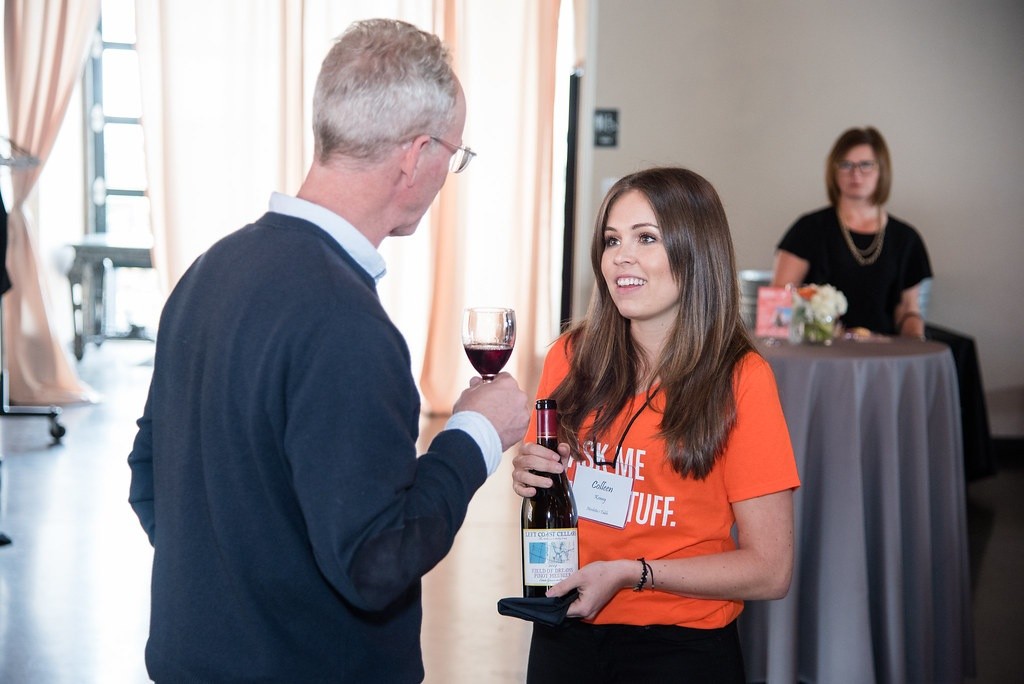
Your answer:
[462,306,516,383]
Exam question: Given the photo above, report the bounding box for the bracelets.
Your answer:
[897,312,923,333]
[633,556,656,592]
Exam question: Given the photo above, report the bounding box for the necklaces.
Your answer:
[838,205,885,266]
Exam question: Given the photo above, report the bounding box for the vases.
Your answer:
[802,322,844,345]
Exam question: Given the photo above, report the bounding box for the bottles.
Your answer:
[521,398,580,598]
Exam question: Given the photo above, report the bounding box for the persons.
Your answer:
[513,167,801,684]
[127,21,531,684]
[769,128,997,483]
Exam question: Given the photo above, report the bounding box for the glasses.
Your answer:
[403,133,477,174]
[834,159,880,174]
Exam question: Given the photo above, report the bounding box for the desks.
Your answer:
[735,334,976,684]
[73,235,157,359]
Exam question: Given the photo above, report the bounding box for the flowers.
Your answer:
[788,284,849,325]
